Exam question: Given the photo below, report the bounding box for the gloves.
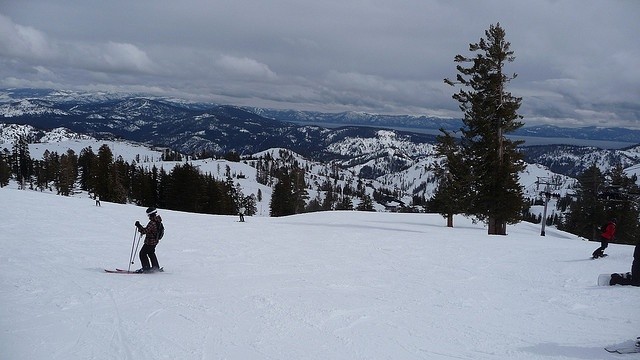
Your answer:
[135,221,141,228]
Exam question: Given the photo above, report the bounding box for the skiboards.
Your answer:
[104,267,168,273]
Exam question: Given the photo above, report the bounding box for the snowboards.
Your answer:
[590,254,609,258]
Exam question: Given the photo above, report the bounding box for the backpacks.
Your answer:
[146,217,164,240]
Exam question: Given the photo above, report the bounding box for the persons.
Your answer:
[96,194,100,207]
[135,207,164,272]
[610,239,640,287]
[239,207,246,222]
[593,218,617,259]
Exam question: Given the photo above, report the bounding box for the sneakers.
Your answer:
[151,266,159,270]
[140,267,150,272]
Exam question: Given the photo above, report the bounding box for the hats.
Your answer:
[146,207,157,215]
